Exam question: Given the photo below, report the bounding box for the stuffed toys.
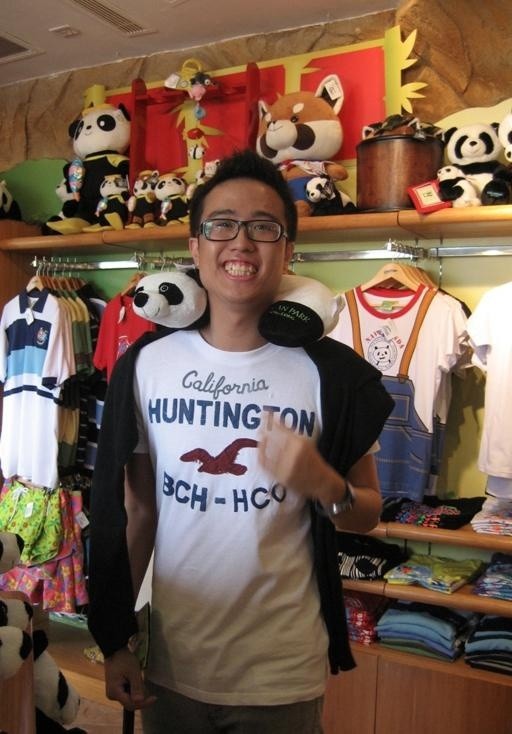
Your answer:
[0,529,88,734]
[255,73,357,218]
[436,110,512,209]
[1,179,20,222]
[126,159,224,230]
[40,103,132,236]
[0,58,358,236]
[131,268,346,348]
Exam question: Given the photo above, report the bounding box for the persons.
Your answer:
[88,149,396,733]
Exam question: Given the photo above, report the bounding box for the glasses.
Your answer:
[199,218,289,242]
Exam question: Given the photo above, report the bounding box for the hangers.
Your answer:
[25,258,85,293]
[359,239,437,293]
[120,253,172,296]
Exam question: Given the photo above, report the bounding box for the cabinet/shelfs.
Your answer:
[0,205,512,734]
